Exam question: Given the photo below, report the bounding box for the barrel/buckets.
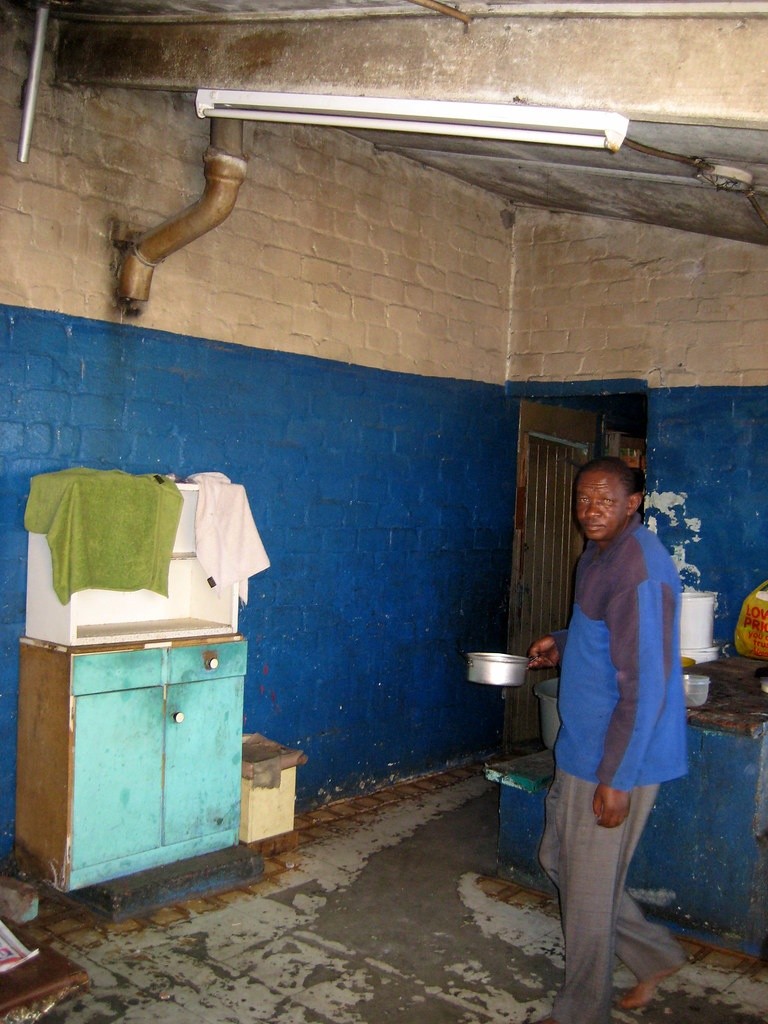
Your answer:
[680,592,718,666]
[534,677,562,749]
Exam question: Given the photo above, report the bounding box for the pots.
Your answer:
[466,652,538,686]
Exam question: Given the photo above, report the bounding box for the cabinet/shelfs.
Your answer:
[15,630,249,896]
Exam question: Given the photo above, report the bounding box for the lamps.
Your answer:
[192,89,628,155]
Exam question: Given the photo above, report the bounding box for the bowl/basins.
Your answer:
[681,675,711,707]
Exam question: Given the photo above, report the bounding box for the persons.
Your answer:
[527,457,689,1024]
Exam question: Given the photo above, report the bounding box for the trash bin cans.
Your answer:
[238,734,303,845]
